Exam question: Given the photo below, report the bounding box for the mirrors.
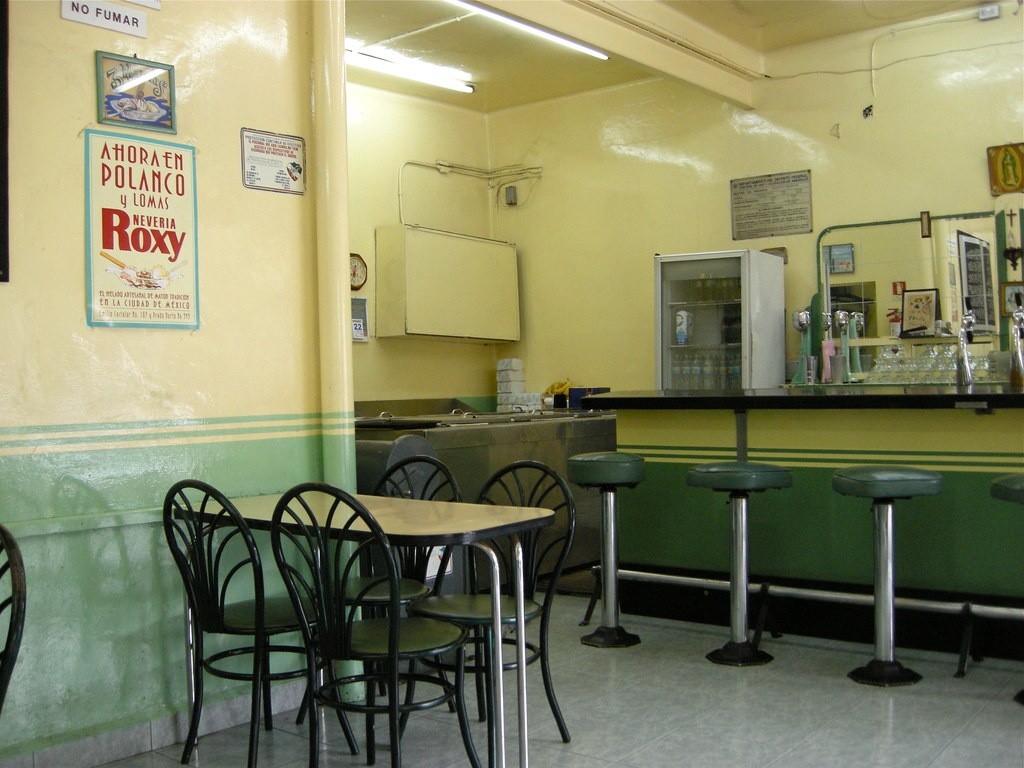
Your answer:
[817,207,1000,386]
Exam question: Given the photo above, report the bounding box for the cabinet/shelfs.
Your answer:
[373,222,521,345]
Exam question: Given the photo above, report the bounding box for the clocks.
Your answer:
[350,253,367,290]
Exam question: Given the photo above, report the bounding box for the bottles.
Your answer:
[671,352,742,389]
[693,273,740,302]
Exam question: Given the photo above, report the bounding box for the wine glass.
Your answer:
[866,344,989,383]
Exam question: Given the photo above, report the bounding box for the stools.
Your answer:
[567,451,645,649]
[686,461,793,667]
[989,473,1024,705]
[832,466,943,687]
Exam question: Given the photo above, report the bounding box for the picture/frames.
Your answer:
[96,51,177,135]
[899,287,940,337]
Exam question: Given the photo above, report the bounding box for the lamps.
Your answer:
[344,37,474,94]
[1002,229,1022,271]
[444,0,611,62]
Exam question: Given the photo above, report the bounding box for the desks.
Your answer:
[173,490,556,768]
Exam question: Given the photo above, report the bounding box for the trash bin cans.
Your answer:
[355,429,472,682]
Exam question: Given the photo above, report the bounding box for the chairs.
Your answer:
[0,523,26,715]
[163,454,577,768]
